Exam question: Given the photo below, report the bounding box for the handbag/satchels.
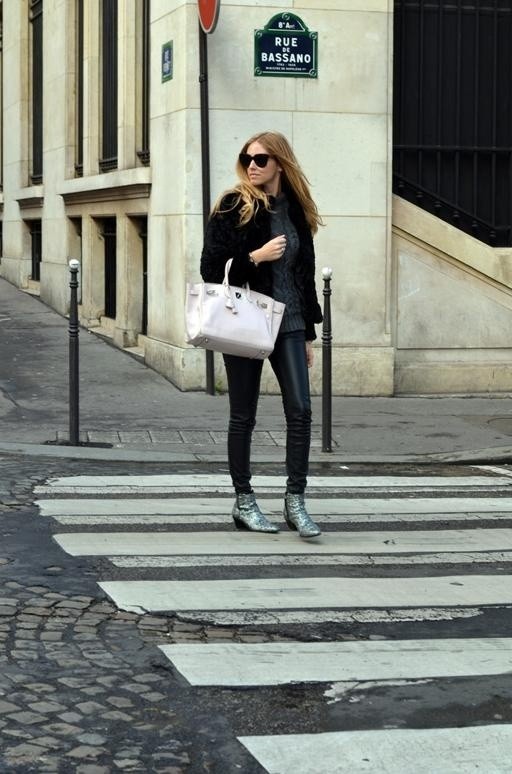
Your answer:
[186,282,286,360]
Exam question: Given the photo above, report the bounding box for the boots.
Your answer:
[232,491,280,533]
[283,492,321,538]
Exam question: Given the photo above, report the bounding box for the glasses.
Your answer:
[239,153,276,168]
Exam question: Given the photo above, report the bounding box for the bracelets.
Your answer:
[248,251,259,267]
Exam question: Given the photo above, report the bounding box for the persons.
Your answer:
[198,129,324,539]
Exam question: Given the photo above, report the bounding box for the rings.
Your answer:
[281,248,284,252]
